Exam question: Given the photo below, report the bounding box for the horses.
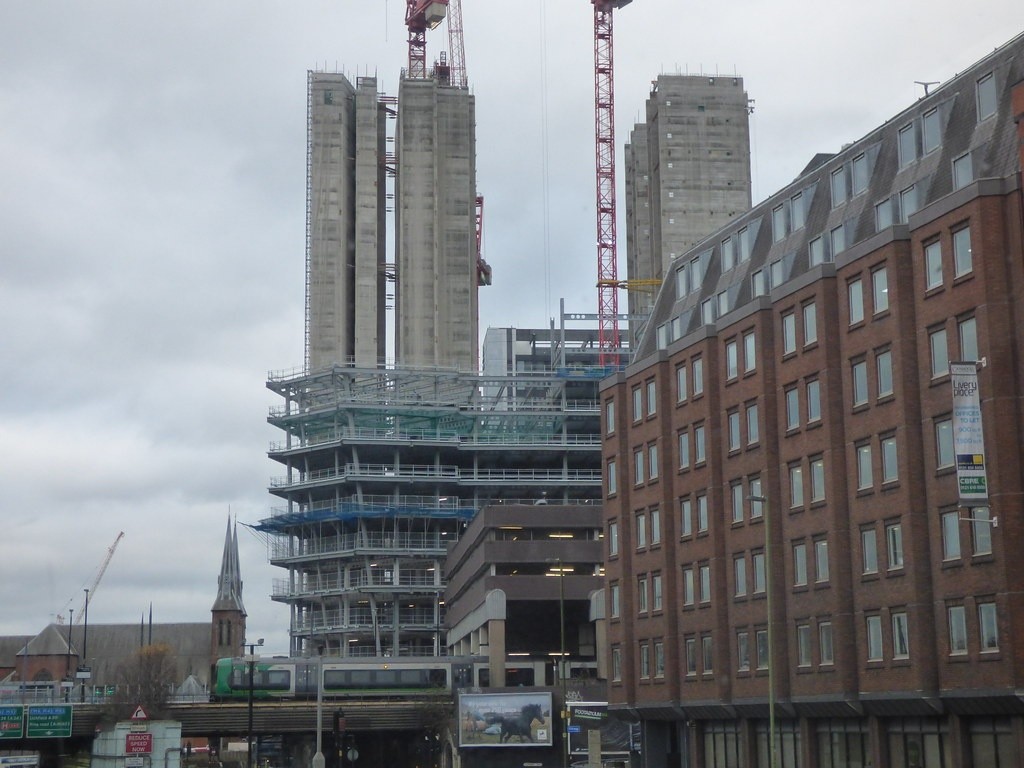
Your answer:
[462,703,545,744]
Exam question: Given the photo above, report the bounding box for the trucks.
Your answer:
[182,746,216,756]
[227,742,257,752]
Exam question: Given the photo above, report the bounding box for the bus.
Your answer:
[207,655,455,707]
[0,754,41,768]
[0,677,76,706]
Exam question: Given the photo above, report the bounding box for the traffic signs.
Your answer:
[0,703,24,739]
[27,705,73,736]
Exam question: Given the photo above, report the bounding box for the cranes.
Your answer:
[56,531,124,625]
[592,0,662,367]
[403,0,493,287]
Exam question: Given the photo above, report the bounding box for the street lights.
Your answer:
[22,639,29,703]
[81,585,93,705]
[545,562,578,768]
[65,609,75,703]
[239,636,265,768]
[311,643,333,768]
[744,492,785,767]
